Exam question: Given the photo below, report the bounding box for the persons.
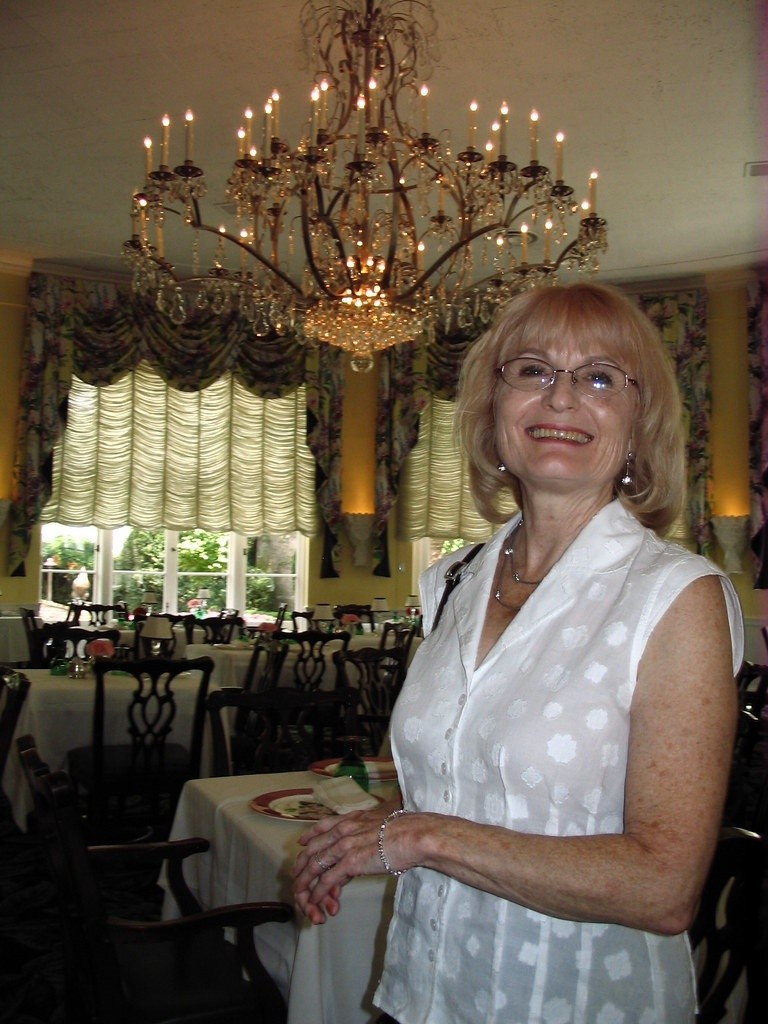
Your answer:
[286,284,747,1023]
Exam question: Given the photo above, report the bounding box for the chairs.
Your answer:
[0,604,767,1024]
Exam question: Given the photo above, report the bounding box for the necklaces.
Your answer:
[493,518,542,614]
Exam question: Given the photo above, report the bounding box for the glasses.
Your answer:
[495,356,637,399]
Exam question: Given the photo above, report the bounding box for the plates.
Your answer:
[249,788,387,822]
[309,756,398,782]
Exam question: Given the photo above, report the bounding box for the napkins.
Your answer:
[315,776,378,815]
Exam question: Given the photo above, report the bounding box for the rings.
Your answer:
[313,851,332,870]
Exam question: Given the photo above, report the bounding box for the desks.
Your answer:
[183,642,383,744]
[0,615,43,665]
[158,770,404,1023]
[6,668,231,809]
[66,623,206,670]
[325,634,422,676]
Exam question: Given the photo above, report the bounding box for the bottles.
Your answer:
[336,747,369,792]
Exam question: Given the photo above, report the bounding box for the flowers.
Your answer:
[340,614,361,625]
[258,622,277,634]
[189,599,201,608]
[85,639,114,658]
[407,606,423,621]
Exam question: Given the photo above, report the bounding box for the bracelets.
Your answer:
[378,809,412,876]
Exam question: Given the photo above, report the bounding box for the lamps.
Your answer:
[131,0,609,374]
[310,604,334,632]
[370,599,389,636]
[404,594,421,607]
[140,592,158,605]
[140,617,173,656]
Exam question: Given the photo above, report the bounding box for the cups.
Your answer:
[50,657,68,675]
